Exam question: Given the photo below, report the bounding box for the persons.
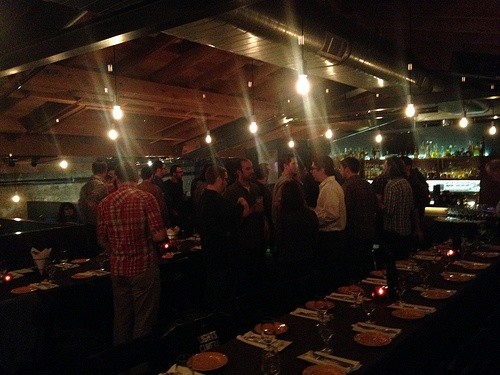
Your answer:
[151,160,169,204]
[57,203,78,223]
[96,162,168,345]
[340,157,374,261]
[399,157,429,245]
[136,167,169,225]
[196,165,249,275]
[376,157,414,267]
[105,168,118,194]
[272,154,317,263]
[224,160,272,260]
[78,160,109,223]
[310,156,347,269]
[255,163,274,258]
[165,165,184,197]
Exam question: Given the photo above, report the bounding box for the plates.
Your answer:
[11,288,37,294]
[255,322,289,335]
[71,274,89,279]
[444,274,472,281]
[392,310,425,319]
[302,364,346,375]
[71,258,91,264]
[187,352,228,371]
[354,332,392,346]
[421,291,447,298]
[306,300,335,309]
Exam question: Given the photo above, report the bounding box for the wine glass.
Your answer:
[60,251,68,271]
[318,321,334,352]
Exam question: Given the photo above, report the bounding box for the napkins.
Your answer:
[30,247,53,275]
[167,225,180,250]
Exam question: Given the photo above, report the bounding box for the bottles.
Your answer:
[344,137,485,180]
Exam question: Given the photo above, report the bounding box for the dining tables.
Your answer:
[0,236,223,375]
[154,240,500,375]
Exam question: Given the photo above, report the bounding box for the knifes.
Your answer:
[313,352,354,368]
[358,324,397,334]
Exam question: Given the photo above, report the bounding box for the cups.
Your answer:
[265,351,281,375]
[30,258,49,283]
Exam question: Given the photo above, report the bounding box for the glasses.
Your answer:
[312,167,318,171]
